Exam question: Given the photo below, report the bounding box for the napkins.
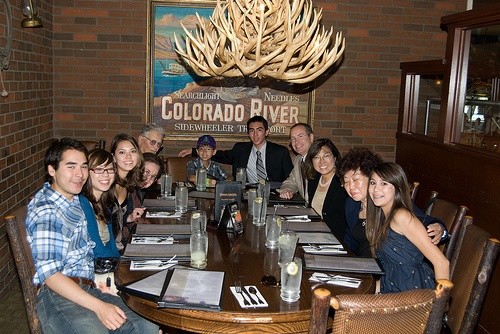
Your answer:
[129,236,175,244]
[229,285,269,309]
[301,244,347,255]
[309,270,362,289]
[145,210,181,219]
[279,215,312,223]
[128,259,178,272]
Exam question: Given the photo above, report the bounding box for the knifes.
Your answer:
[241,286,257,305]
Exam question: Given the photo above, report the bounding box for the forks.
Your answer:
[317,277,362,283]
[307,243,344,250]
[235,281,250,305]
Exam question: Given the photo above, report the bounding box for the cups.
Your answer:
[236,167,246,189]
[191,210,207,231]
[265,216,283,250]
[259,181,270,204]
[253,197,266,226]
[196,169,207,191]
[174,186,188,212]
[248,188,262,215]
[280,257,303,303]
[190,230,209,269]
[161,174,172,195]
[277,230,298,266]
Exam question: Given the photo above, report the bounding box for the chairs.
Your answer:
[425,190,473,257]
[307,276,454,334]
[160,154,199,185]
[444,215,500,334]
[3,194,44,334]
[408,179,421,205]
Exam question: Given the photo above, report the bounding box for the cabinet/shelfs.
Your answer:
[394,2,500,334]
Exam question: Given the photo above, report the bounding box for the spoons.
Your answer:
[249,287,264,304]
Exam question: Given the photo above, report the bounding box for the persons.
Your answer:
[88,122,165,292]
[304,138,348,239]
[365,162,450,311]
[178,115,294,188]
[25,137,162,334]
[279,122,314,203]
[337,148,448,258]
[186,135,228,183]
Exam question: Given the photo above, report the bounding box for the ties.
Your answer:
[256,151,266,181]
[300,157,306,196]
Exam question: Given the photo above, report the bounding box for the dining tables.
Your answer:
[114,184,379,334]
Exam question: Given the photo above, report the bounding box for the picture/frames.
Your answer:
[146,0,316,143]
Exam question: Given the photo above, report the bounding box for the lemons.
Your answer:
[286,262,298,275]
[277,218,281,227]
[192,213,200,219]
[259,180,266,184]
[279,235,288,245]
[255,197,263,203]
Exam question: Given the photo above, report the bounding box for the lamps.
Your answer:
[20,0,43,28]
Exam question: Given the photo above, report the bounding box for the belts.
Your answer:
[36,277,95,292]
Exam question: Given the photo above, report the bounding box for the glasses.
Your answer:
[142,136,164,149]
[311,153,333,163]
[88,167,116,174]
[290,133,309,142]
[143,170,157,182]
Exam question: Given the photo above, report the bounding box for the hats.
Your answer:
[198,135,216,147]
